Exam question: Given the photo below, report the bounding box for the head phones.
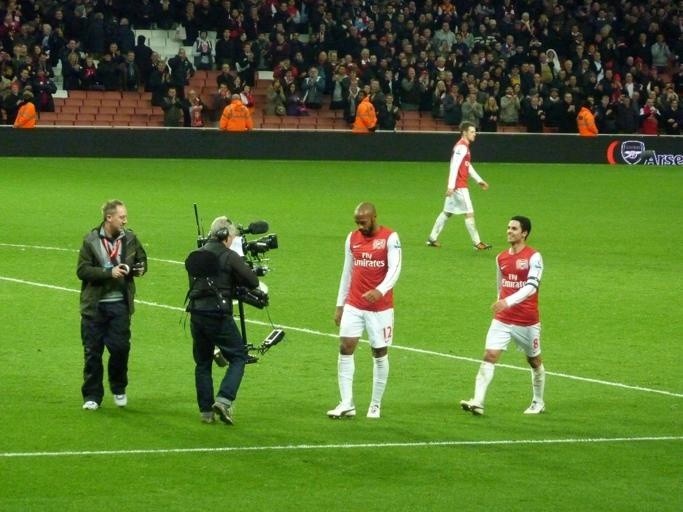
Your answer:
[216,228,229,241]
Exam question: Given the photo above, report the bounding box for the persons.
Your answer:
[184,213,260,426]
[2,0,681,141]
[326,201,402,420]
[425,120,493,250]
[76,199,148,411]
[460,214,546,417]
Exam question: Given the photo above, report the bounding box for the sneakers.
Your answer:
[460,399,484,416]
[473,242,491,250]
[425,239,442,247]
[327,403,356,418]
[202,413,216,423]
[83,401,99,411]
[115,394,127,407]
[368,405,380,419]
[212,401,235,424]
[523,401,545,414]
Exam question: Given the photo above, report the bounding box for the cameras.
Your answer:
[120,262,145,275]
